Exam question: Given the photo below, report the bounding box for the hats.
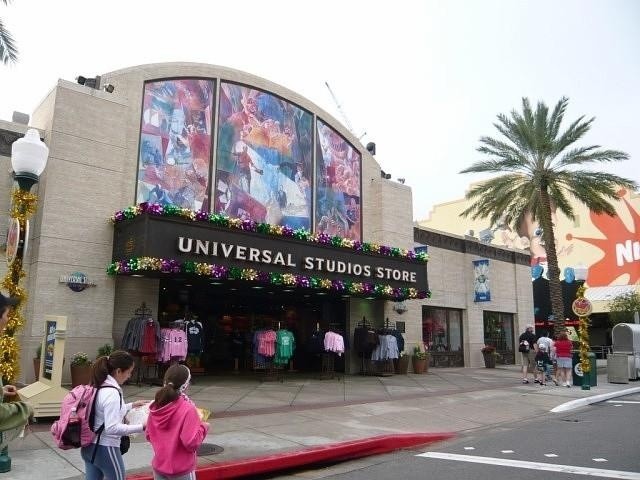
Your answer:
[526,324,532,329]
[538,344,545,349]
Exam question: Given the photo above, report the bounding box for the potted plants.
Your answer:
[33,344,46,379]
[480,346,501,368]
[413,339,432,374]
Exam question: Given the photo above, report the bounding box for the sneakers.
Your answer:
[522,378,571,388]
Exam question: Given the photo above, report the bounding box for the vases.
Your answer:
[70,363,90,389]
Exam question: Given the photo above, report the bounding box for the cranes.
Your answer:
[324,80,367,141]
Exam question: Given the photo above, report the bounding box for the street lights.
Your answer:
[575,262,590,391]
[3,128,49,383]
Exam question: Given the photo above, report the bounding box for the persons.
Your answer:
[278,183,288,213]
[81,350,147,480]
[518,323,573,388]
[235,144,265,193]
[0,291,35,478]
[145,364,210,480]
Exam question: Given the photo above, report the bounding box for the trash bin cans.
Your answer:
[571,350,597,390]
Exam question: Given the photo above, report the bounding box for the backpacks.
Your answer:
[51,381,122,449]
[536,360,547,372]
[519,335,529,352]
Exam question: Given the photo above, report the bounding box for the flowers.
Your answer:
[69,351,96,368]
